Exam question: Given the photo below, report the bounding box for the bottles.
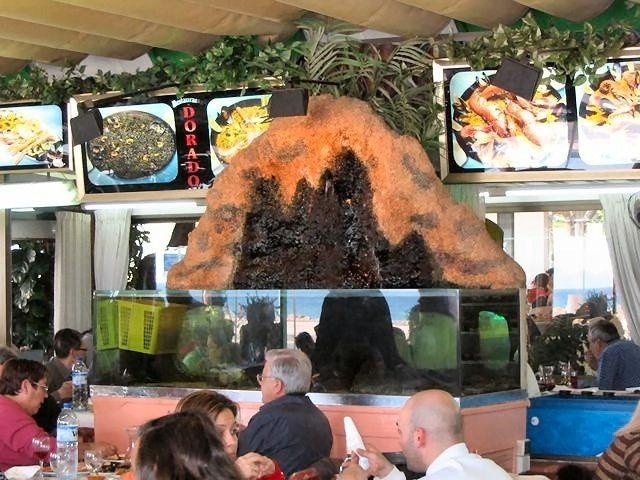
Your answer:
[56,403,80,480]
[71,357,89,412]
[570,372,579,387]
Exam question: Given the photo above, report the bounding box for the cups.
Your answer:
[535,364,555,385]
[83,449,103,480]
[560,361,571,386]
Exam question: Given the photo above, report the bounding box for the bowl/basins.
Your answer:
[211,98,272,167]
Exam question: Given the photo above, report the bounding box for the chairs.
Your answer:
[289,459,335,480]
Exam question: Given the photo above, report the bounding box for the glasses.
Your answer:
[257,374,278,386]
[31,381,49,392]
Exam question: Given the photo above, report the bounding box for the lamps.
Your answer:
[488,44,581,102]
[67,78,195,150]
[263,77,345,121]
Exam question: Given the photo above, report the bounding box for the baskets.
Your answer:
[118,299,187,355]
[96,299,118,352]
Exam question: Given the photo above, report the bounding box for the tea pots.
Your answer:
[124,424,141,468]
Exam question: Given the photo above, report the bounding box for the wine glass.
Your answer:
[32,437,51,472]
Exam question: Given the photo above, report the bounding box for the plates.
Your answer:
[86,109,176,181]
[579,65,638,124]
[42,470,90,477]
[0,110,61,164]
[453,72,567,165]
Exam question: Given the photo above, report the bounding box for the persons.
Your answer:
[175,389,287,480]
[589,395,640,480]
[0,359,119,476]
[42,327,85,401]
[336,390,513,480]
[0,345,18,379]
[109,324,318,387]
[526,272,552,306]
[546,268,554,305]
[126,409,246,480]
[235,347,333,480]
[585,318,640,391]
